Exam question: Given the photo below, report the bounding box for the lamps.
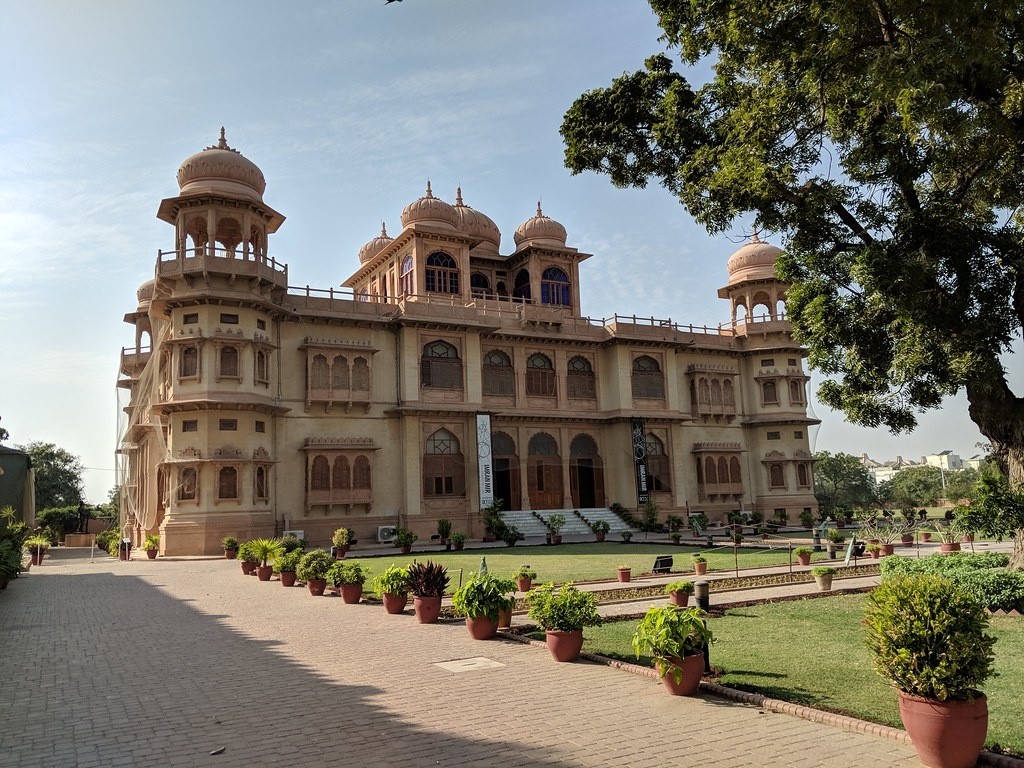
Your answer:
[653,555,673,572]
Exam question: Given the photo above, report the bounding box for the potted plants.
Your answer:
[513,564,538,592]
[117,543,133,560]
[399,559,451,623]
[332,527,352,559]
[621,532,633,542]
[730,516,744,535]
[369,563,411,615]
[452,531,466,550]
[813,520,976,558]
[693,556,707,576]
[452,571,517,640]
[238,537,369,607]
[24,537,50,565]
[483,517,499,543]
[860,572,1000,767]
[393,528,417,554]
[670,518,680,532]
[501,525,519,547]
[631,604,717,696]
[437,519,451,544]
[672,534,680,544]
[592,521,611,542]
[617,564,631,582]
[810,567,837,590]
[221,537,238,559]
[522,581,604,662]
[732,534,743,547]
[143,535,160,559]
[793,546,813,566]
[548,514,566,544]
[663,581,693,607]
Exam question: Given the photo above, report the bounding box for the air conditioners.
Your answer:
[282,530,305,541]
[376,525,397,544]
